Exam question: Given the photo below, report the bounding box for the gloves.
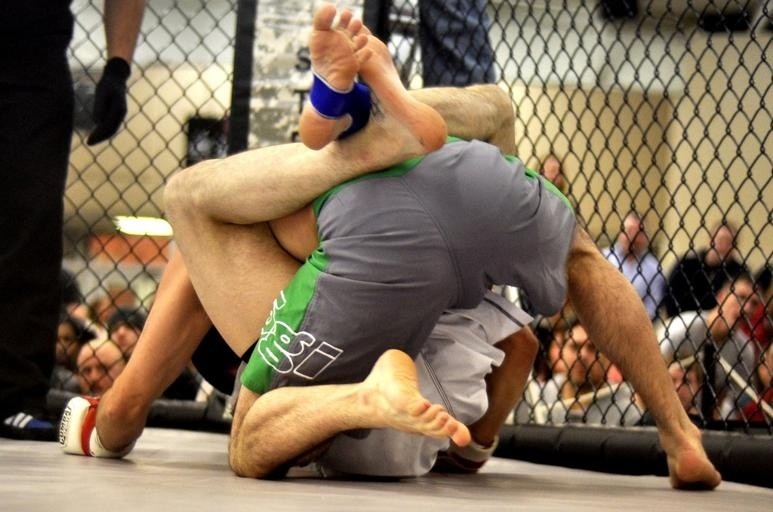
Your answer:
[87,57,130,146]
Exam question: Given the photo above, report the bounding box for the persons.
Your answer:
[506,151,773,436]
[0,0,148,441]
[162,2,541,484]
[51,272,203,400]
[57,131,724,491]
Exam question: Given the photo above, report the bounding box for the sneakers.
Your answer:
[0,412,57,442]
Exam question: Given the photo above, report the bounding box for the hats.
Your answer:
[109,308,145,339]
